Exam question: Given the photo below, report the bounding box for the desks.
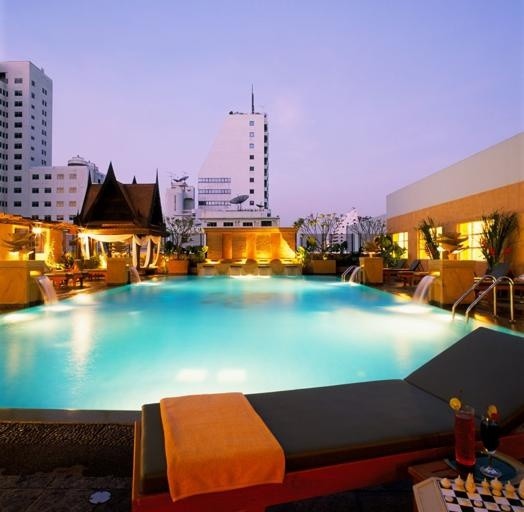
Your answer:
[403,441,523,512]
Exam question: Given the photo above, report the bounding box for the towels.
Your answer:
[157,391,287,503]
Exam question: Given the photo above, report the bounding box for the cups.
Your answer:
[456,406,475,465]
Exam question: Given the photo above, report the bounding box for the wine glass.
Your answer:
[480,416,501,478]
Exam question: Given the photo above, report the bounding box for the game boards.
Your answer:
[412,476,523,512]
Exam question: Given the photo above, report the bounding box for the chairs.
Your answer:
[125,326,523,512]
[382,255,446,291]
[474,261,524,316]
[21,248,162,291]
